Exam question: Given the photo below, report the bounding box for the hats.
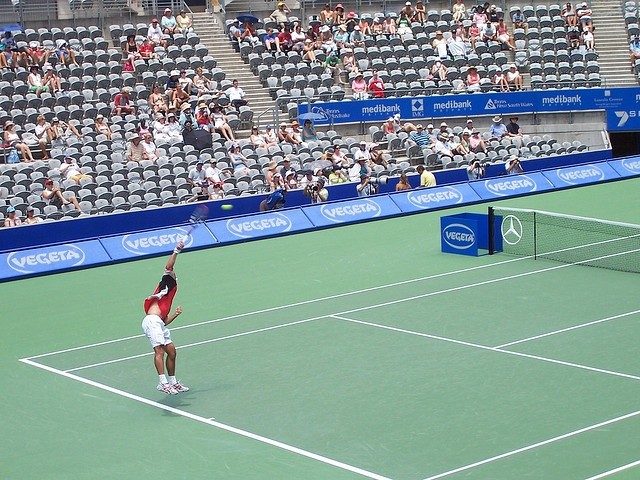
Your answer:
[322,25,330,33]
[471,129,480,135]
[285,170,295,180]
[583,27,589,32]
[7,206,15,213]
[582,2,587,7]
[168,113,175,118]
[335,4,344,11]
[281,122,286,127]
[304,120,312,126]
[211,95,219,101]
[27,206,34,212]
[125,89,132,95]
[58,41,66,49]
[164,8,172,15]
[510,155,518,161]
[440,122,447,128]
[129,133,143,140]
[231,144,240,150]
[277,2,284,6]
[492,116,502,122]
[496,68,501,72]
[196,160,204,166]
[46,66,52,70]
[510,116,518,118]
[439,133,448,140]
[44,179,53,185]
[354,25,361,31]
[347,12,356,18]
[327,148,334,154]
[466,120,472,123]
[448,133,454,137]
[185,119,192,124]
[291,121,299,128]
[355,73,364,79]
[212,104,223,111]
[387,119,393,122]
[210,159,218,163]
[417,124,422,129]
[405,1,412,6]
[268,161,277,170]
[360,174,369,180]
[180,9,186,14]
[5,120,14,128]
[452,29,456,33]
[317,179,325,185]
[30,66,38,72]
[30,43,37,48]
[52,118,59,122]
[180,70,186,73]
[572,29,576,33]
[266,126,272,129]
[510,64,516,69]
[359,141,366,144]
[313,168,322,176]
[436,30,443,35]
[331,165,341,172]
[198,103,207,110]
[152,18,158,23]
[427,124,433,129]
[634,34,639,39]
[96,114,103,120]
[330,51,336,56]
[272,172,280,180]
[202,183,209,188]
[127,33,135,40]
[370,143,379,150]
[468,67,477,72]
[385,14,390,18]
[325,3,330,7]
[308,26,312,30]
[373,17,380,23]
[157,115,164,120]
[358,157,368,160]
[338,24,347,31]
[305,169,312,175]
[417,0,422,3]
[283,157,290,161]
[361,15,365,19]
[306,39,311,43]
[434,59,442,63]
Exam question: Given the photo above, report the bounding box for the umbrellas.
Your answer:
[236,15,258,23]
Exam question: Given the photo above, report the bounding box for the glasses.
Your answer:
[253,129,257,130]
[336,148,340,149]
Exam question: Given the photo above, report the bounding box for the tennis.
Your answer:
[220,205,233,210]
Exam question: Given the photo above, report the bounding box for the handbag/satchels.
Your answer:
[7,149,21,163]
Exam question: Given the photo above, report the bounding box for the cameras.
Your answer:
[515,160,521,165]
[312,32,317,43]
[476,162,490,167]
[202,110,209,117]
[276,24,283,33]
[368,176,388,185]
[307,184,319,191]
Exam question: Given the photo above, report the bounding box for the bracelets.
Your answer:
[173,247,181,254]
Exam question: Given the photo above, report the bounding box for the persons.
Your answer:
[452,0,466,23]
[22,206,43,226]
[368,69,385,98]
[142,241,190,395]
[301,170,318,187]
[250,125,267,148]
[3,120,33,163]
[303,119,318,141]
[580,26,595,50]
[43,179,82,213]
[140,133,160,159]
[561,3,578,28]
[304,177,329,203]
[240,20,259,45]
[221,80,250,107]
[332,144,345,163]
[467,2,498,22]
[568,29,580,49]
[396,174,412,191]
[148,19,167,48]
[431,61,449,80]
[437,122,449,142]
[283,170,297,189]
[329,165,347,183]
[35,113,53,159]
[354,140,375,169]
[505,155,523,174]
[448,134,467,154]
[278,122,302,145]
[147,68,220,112]
[262,2,427,71]
[469,129,488,154]
[314,168,329,185]
[463,119,475,135]
[175,9,194,33]
[59,156,92,183]
[407,124,435,152]
[208,184,225,200]
[188,161,208,186]
[139,38,158,59]
[482,21,497,41]
[513,10,529,39]
[506,116,522,137]
[467,159,485,180]
[383,113,417,134]
[260,189,288,211]
[431,30,448,59]
[4,206,22,228]
[356,175,378,196]
[114,87,136,116]
[629,37,640,67]
[447,29,466,45]
[349,157,371,181]
[493,68,510,92]
[577,2,593,32]
[269,173,285,191]
[490,116,508,138]
[426,124,437,139]
[227,143,248,169]
[263,125,278,145]
[187,184,211,202]
[351,73,367,95]
[321,148,335,162]
[161,8,179,34]
[280,158,297,179]
[125,34,143,72]
[29,65,49,95]
[168,112,236,146]
[135,119,149,139]
[465,66,482,93]
[93,114,112,140]
[49,117,84,140]
[468,23,479,49]
[44,66,65,95]
[435,132,454,160]
[368,142,388,166]
[126,133,147,160]
[266,161,282,183]
[290,120,303,143]
[416,166,437,186]
[458,132,470,153]
[496,19,515,50]
[152,112,171,151]
[205,158,232,185]
[229,20,243,44]
[0,29,78,65]
[506,64,524,91]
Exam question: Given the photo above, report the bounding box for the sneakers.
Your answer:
[171,380,189,392]
[156,381,178,395]
